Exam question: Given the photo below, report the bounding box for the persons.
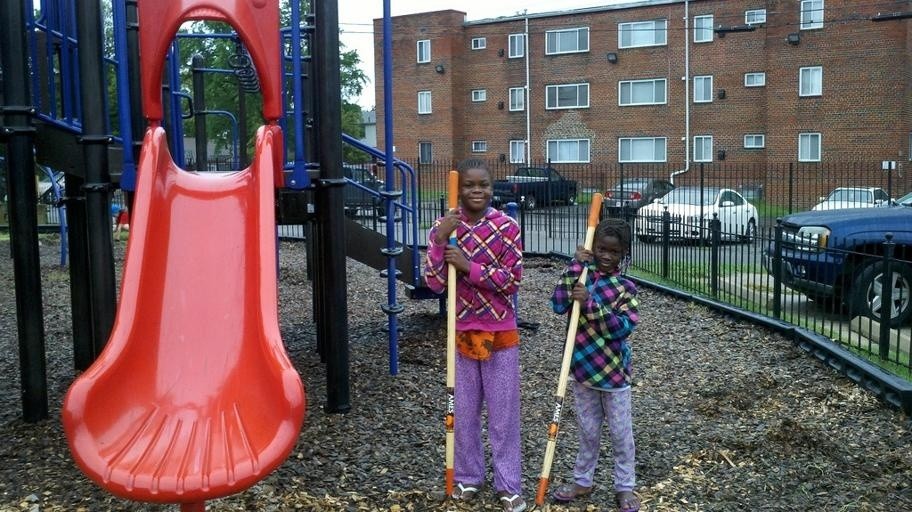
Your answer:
[112,204,130,241]
[424,157,527,512]
[553,218,641,512]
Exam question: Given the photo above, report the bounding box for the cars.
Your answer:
[603,175,677,216]
[809,183,893,211]
[633,182,761,247]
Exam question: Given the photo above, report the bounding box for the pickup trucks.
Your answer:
[491,165,579,210]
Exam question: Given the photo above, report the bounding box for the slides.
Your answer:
[60,123,306,509]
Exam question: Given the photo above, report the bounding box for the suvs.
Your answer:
[757,187,912,329]
[342,162,396,218]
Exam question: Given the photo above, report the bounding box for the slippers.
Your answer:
[554,483,578,501]
[451,482,481,502]
[500,494,527,512]
[619,496,642,512]
